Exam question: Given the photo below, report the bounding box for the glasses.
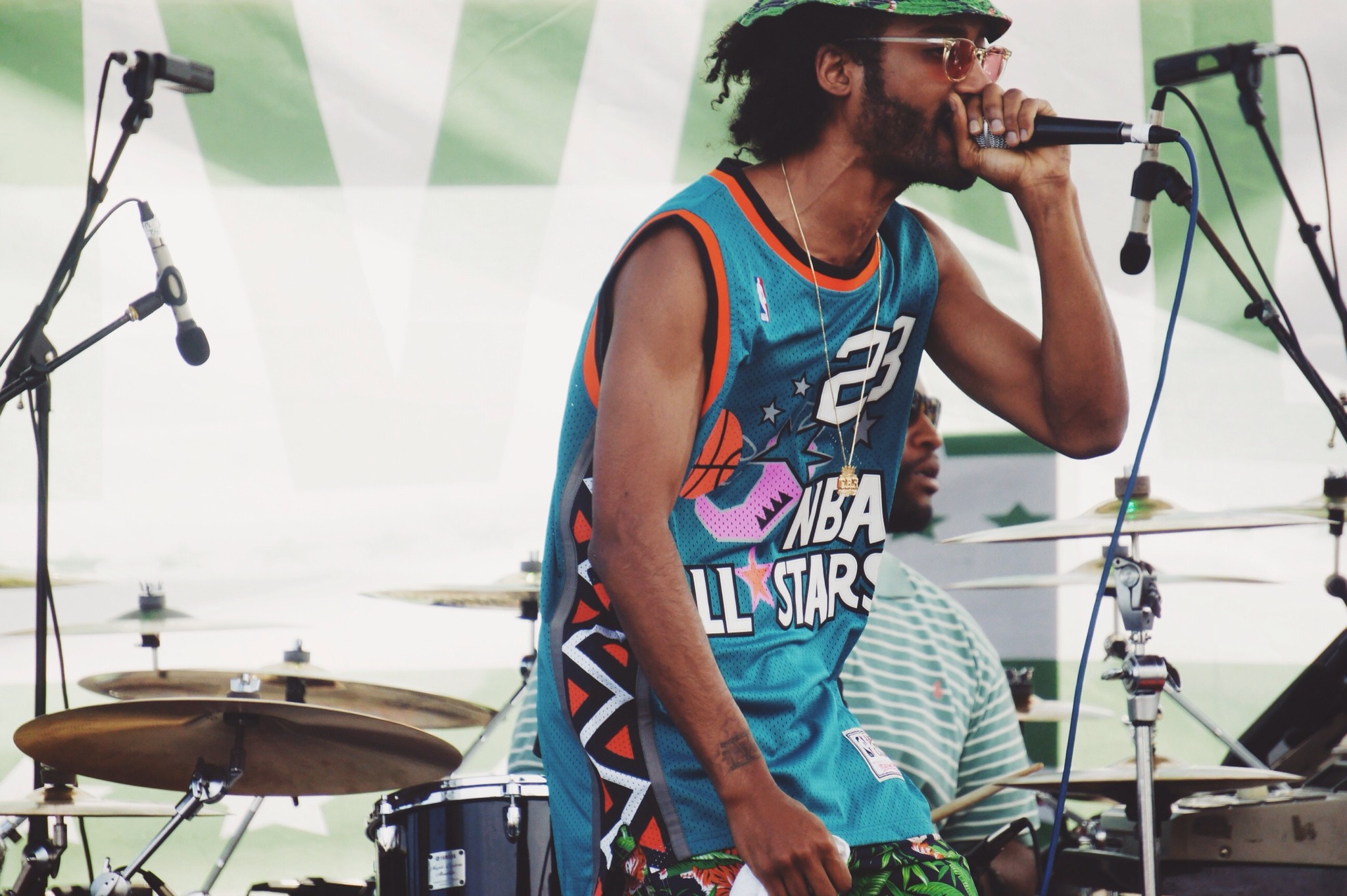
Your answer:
[909,391,942,427]
[860,37,1012,85]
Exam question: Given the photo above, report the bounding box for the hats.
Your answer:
[738,0,1010,43]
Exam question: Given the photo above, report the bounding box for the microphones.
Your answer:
[114,49,218,94]
[1153,40,1300,88]
[968,113,1180,154]
[1120,91,1167,275]
[138,200,214,367]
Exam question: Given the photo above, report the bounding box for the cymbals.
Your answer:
[942,555,1288,587]
[0,797,237,819]
[73,669,499,729]
[11,696,465,798]
[0,573,91,593]
[991,757,1307,786]
[352,583,543,615]
[1016,693,1122,726]
[2,606,306,642]
[940,497,1343,546]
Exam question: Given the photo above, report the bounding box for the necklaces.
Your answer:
[778,156,882,495]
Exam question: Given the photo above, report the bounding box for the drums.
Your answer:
[365,772,563,896]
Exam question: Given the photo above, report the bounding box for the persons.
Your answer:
[537,0,1130,896]
[508,372,1042,896]
